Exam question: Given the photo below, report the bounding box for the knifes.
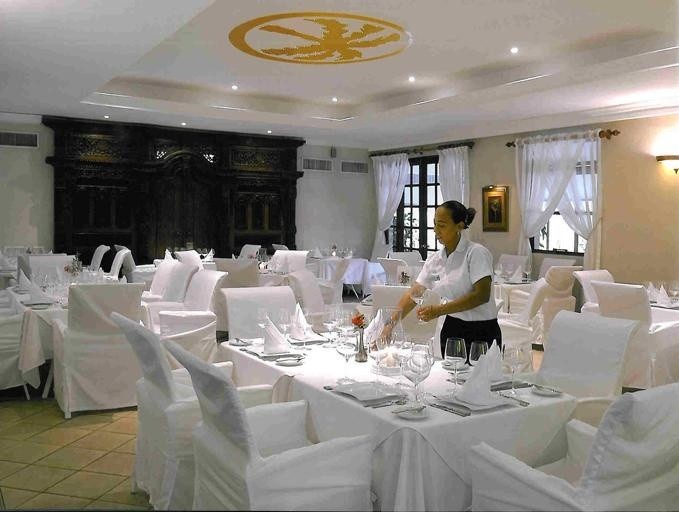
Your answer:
[533,383,562,394]
[430,403,471,418]
[234,336,247,344]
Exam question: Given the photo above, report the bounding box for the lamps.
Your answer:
[656,155,679,174]
[488,185,497,189]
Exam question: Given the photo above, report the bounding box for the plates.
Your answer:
[228,339,253,347]
[531,383,564,396]
[394,406,432,420]
[440,392,516,411]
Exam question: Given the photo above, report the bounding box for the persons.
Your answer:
[489,199,501,222]
[371,199,504,366]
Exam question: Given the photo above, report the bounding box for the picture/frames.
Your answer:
[482,185,509,232]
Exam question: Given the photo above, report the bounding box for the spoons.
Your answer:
[391,406,427,415]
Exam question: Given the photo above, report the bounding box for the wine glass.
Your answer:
[167,247,215,263]
[410,286,429,325]
[493,261,532,284]
[638,279,679,309]
[233,246,282,275]
[253,302,534,407]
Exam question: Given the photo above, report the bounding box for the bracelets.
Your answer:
[385,317,398,328]
